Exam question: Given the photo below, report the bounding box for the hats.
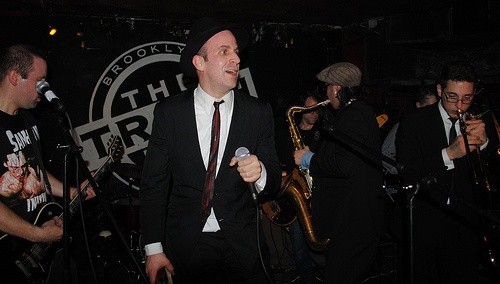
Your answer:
[315,62,361,86]
[179,22,243,70]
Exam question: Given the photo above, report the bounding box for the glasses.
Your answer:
[443,90,474,105]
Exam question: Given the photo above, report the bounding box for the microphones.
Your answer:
[235,147,257,200]
[35,80,66,111]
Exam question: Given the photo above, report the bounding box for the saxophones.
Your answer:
[259,99,331,254]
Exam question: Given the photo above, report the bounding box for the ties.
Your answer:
[201,99,224,221]
[448,117,460,146]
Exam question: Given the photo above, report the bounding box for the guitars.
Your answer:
[129,177,173,284]
[0,134,125,284]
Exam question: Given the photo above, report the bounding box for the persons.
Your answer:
[0,42,99,284]
[279,86,330,188]
[304,62,387,284]
[1,148,49,202]
[140,21,281,284]
[394,62,500,284]
[380,84,442,284]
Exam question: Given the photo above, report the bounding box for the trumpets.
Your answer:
[457,109,500,280]
